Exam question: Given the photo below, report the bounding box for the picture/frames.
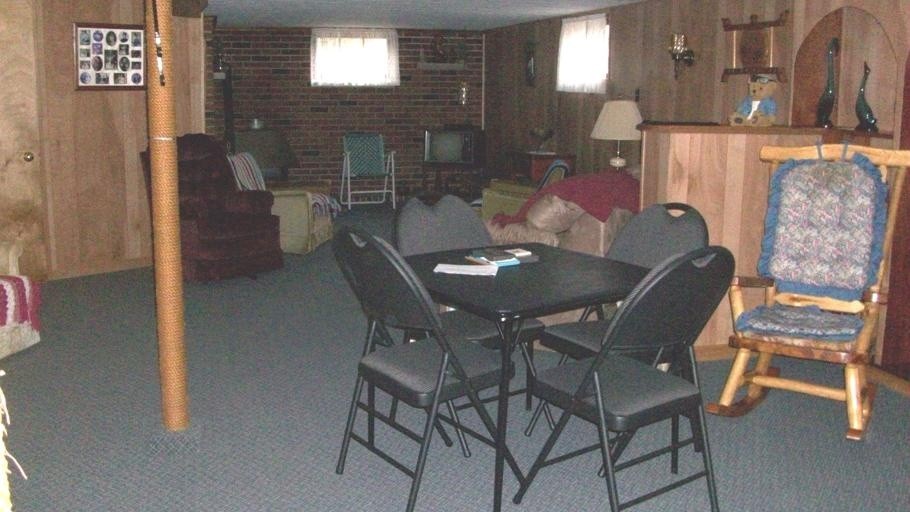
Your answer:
[72,21,145,91]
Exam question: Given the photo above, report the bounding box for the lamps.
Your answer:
[590,99,644,172]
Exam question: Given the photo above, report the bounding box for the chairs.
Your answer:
[705,143,910,443]
[469,159,571,223]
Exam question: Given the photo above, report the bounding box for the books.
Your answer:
[433,247,542,279]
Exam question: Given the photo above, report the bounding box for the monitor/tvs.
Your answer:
[426,129,484,164]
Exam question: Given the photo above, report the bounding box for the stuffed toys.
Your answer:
[729,72,780,131]
